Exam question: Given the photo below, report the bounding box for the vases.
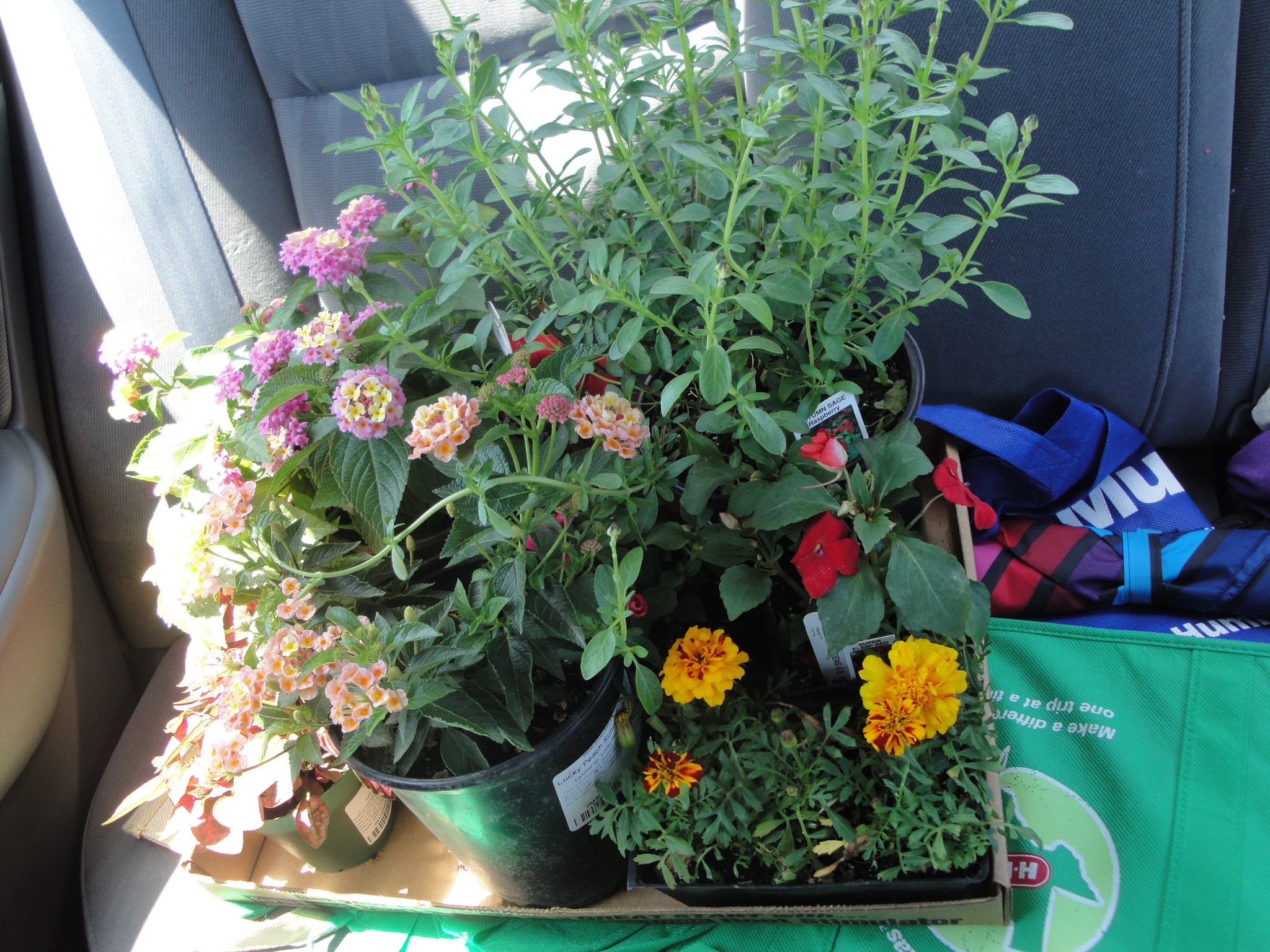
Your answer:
[315,643,631,908]
[251,764,395,877]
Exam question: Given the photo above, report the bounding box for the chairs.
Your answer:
[232,0,1270,646]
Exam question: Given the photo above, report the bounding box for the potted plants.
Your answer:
[325,1,1081,525]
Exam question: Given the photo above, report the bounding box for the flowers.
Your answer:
[96,154,1082,879]
